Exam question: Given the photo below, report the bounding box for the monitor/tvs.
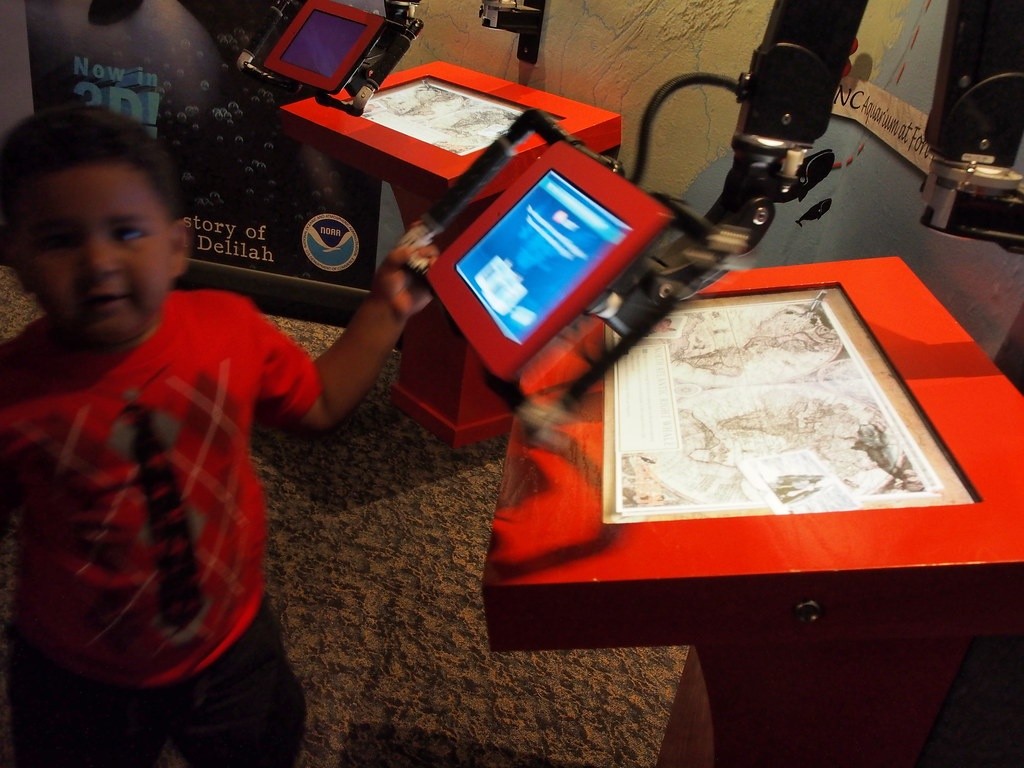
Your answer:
[428,141,671,379]
[264,0,387,95]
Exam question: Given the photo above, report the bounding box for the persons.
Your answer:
[0,104,438,768]
